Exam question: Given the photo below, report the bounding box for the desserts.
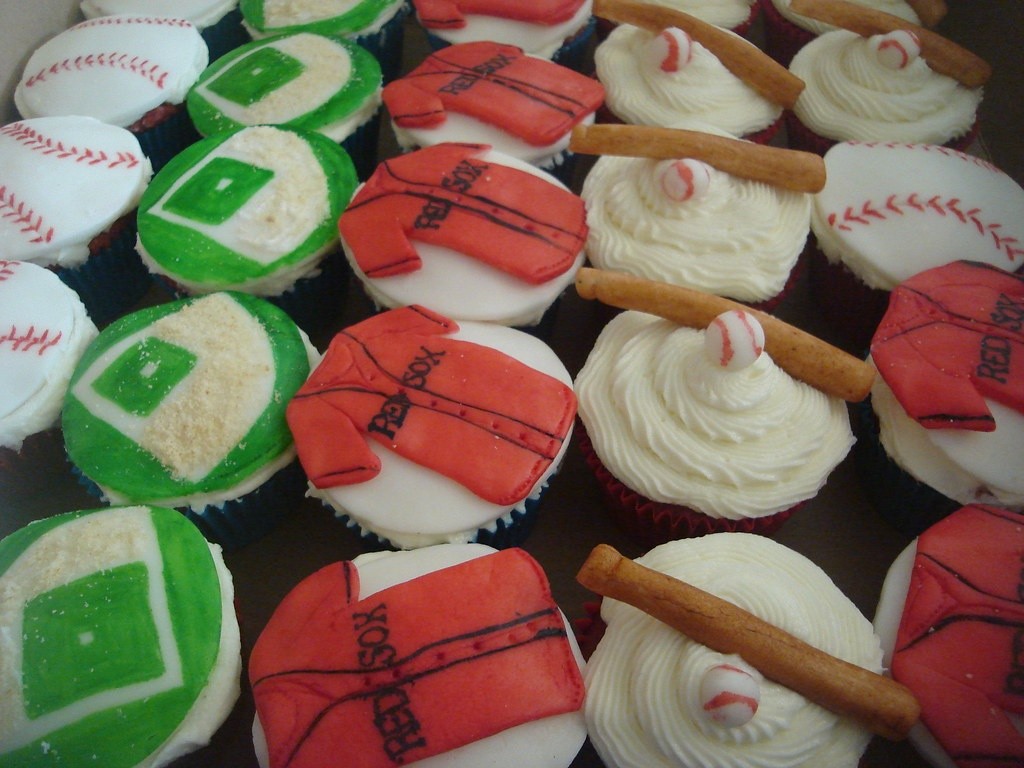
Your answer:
[1,1,1023,768]
